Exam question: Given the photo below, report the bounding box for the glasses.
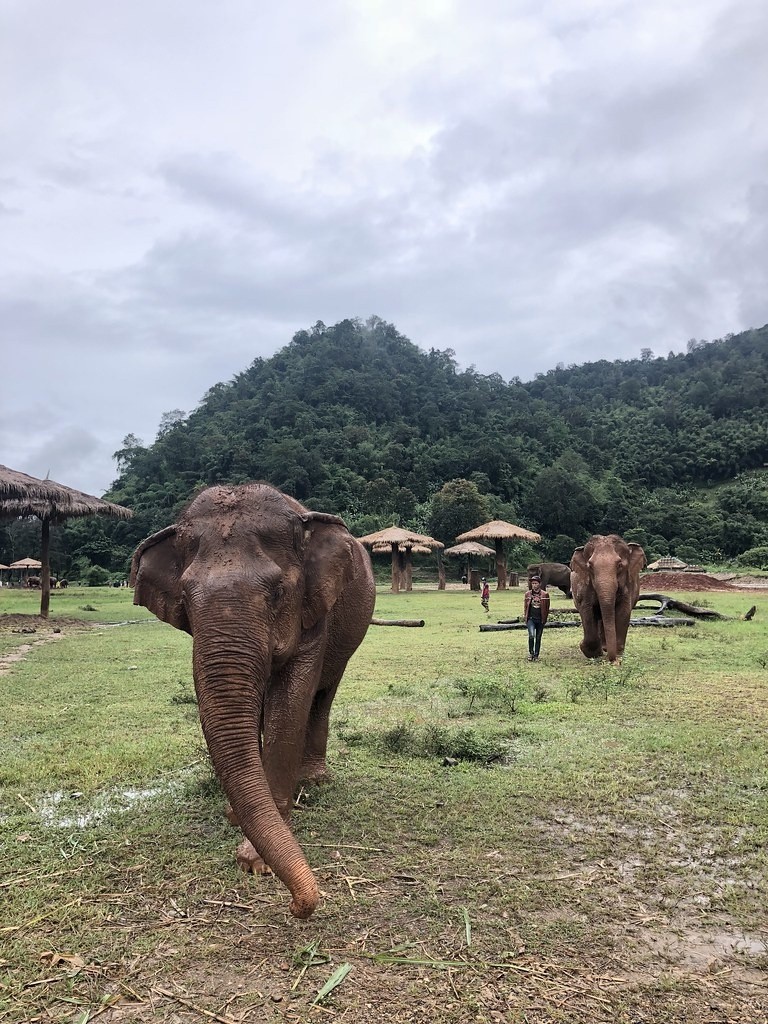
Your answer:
[482,580,484,581]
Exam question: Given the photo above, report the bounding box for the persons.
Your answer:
[524,576,550,661]
[480,577,490,613]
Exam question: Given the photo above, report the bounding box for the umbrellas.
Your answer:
[0,462,131,620]
[356,525,444,594]
[454,520,540,590]
[443,542,495,591]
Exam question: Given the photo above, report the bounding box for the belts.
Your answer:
[532,616,541,620]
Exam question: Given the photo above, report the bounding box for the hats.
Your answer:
[529,576,541,581]
[481,577,486,581]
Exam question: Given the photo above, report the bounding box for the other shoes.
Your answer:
[533,656,539,661]
[484,611,486,613]
[486,609,489,611]
[528,654,533,661]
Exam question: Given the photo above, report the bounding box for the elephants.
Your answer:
[132,479,376,919]
[569,534,647,666]
[527,562,572,599]
